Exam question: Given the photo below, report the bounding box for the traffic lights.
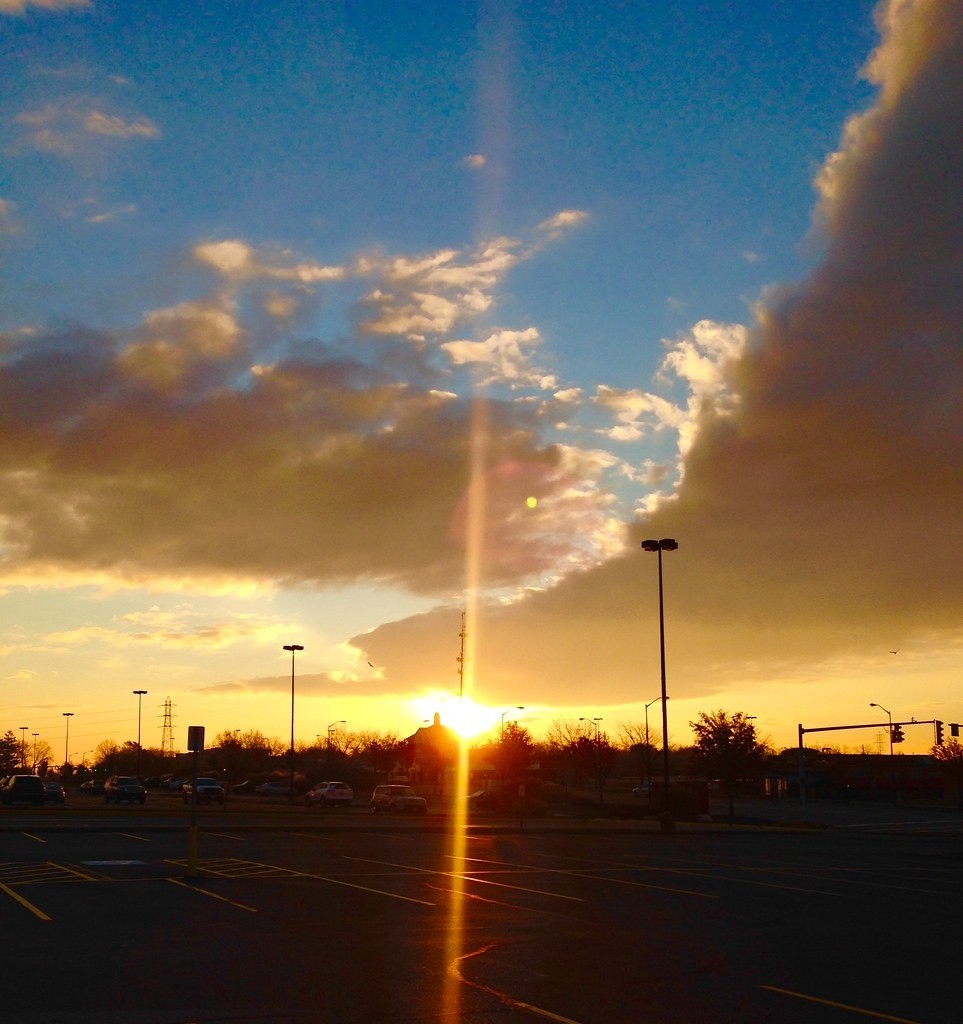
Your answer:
[936,721,944,745]
[84,769,87,772]
[94,769,97,772]
[891,729,905,743]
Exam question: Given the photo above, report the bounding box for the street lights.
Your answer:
[645,696,669,811]
[134,690,148,775]
[20,727,28,770]
[83,750,94,767]
[63,712,73,778]
[869,703,893,791]
[68,753,78,764]
[579,718,596,744]
[642,538,680,817]
[747,716,757,727]
[32,734,39,773]
[502,707,524,740]
[328,721,346,748]
[282,644,305,803]
[317,735,329,747]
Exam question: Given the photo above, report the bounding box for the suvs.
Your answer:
[103,775,147,805]
[43,782,66,806]
[304,782,355,809]
[3,775,46,808]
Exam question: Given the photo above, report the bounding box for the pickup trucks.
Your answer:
[182,778,224,806]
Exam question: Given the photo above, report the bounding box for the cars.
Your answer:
[369,784,426,816]
[232,777,272,794]
[256,782,288,798]
[81,782,90,795]
[632,787,652,793]
[0,776,12,793]
[460,791,495,810]
[87,780,103,797]
